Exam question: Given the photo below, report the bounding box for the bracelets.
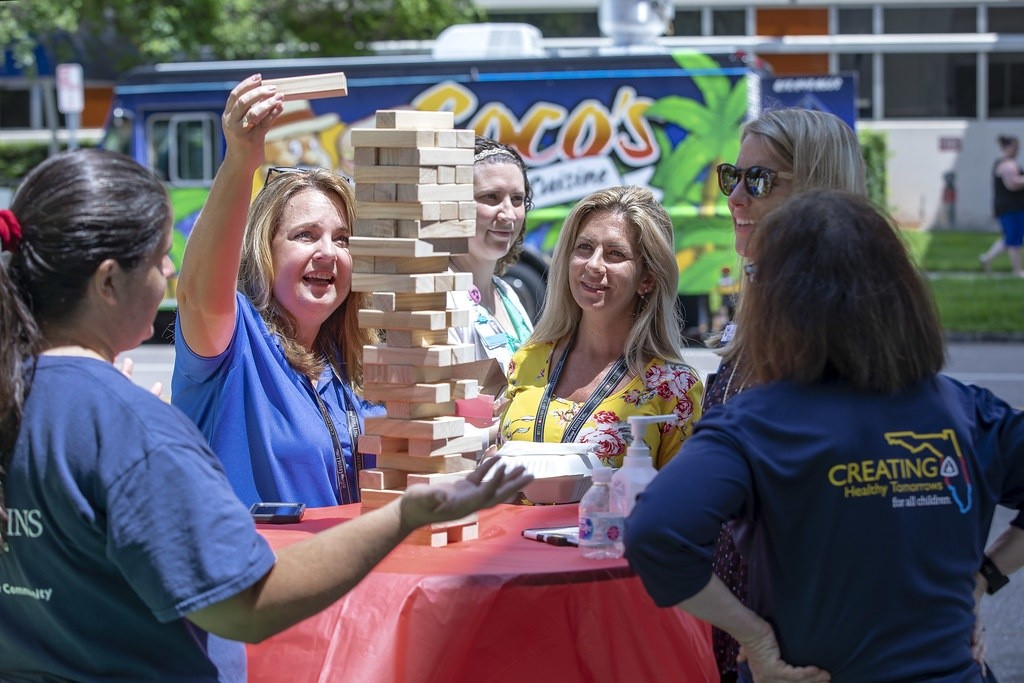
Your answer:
[979,555,1009,596]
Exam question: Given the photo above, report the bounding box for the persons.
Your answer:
[623,187,1023,683]
[978,136,1024,278]
[169,74,386,509]
[701,107,866,682]
[497,185,706,471]
[446,137,533,464]
[0,149,534,682]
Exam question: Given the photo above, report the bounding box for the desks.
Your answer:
[241,500,721,683]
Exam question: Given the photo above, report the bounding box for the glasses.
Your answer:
[264,166,351,191]
[716,163,795,200]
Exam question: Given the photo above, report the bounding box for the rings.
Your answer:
[243,116,252,129]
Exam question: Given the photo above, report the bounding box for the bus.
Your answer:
[76,51,852,343]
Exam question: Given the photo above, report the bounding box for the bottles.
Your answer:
[577,467,625,560]
